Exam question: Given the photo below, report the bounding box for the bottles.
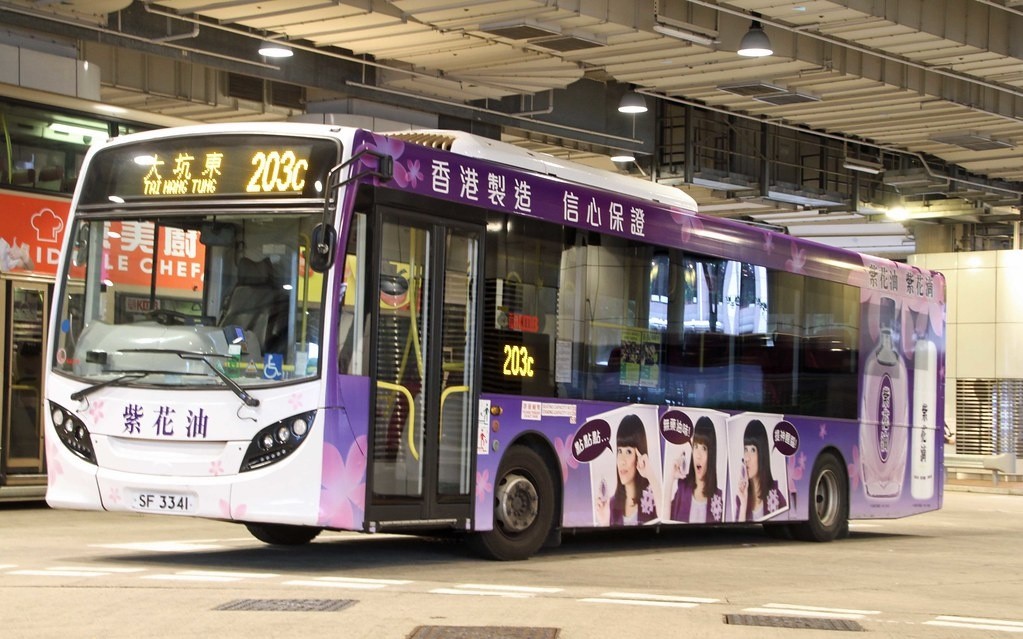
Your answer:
[860,329,909,498]
[910,340,937,501]
[598,476,608,502]
[680,451,687,476]
[740,459,746,480]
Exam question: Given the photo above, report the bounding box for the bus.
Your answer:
[39,120,946,563]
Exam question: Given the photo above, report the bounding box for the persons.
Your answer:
[596,413,659,526]
[663,415,723,522]
[734,420,787,523]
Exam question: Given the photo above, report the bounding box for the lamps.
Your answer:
[653,0,722,46]
[738,11,773,57]
[611,148,635,163]
[842,141,884,175]
[617,84,648,113]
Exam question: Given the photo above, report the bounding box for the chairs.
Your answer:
[597,328,860,418]
[213,284,289,362]
[1,165,78,196]
[258,38,294,59]
